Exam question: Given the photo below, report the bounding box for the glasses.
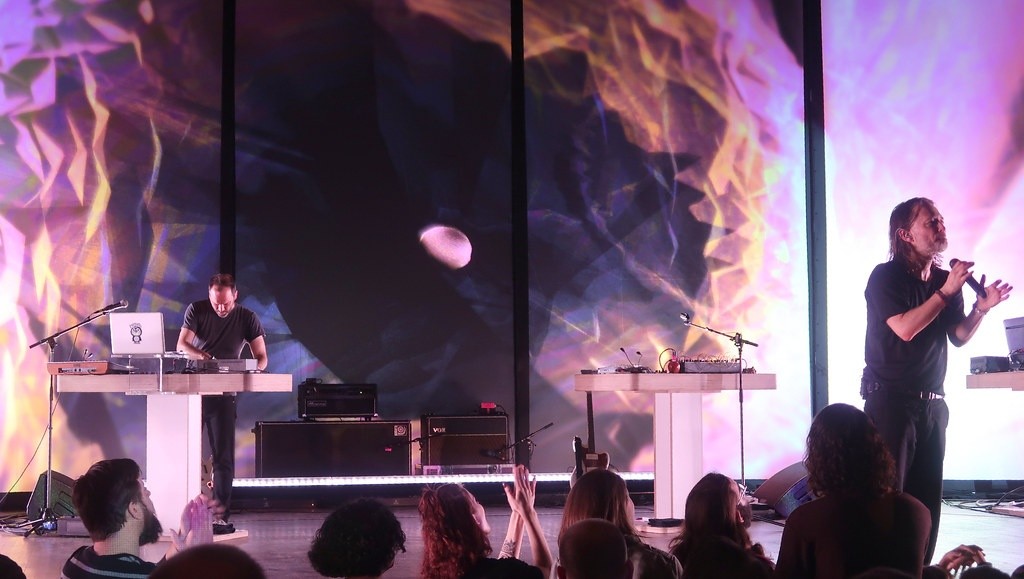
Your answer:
[737,483,746,507]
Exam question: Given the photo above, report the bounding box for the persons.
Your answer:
[860,197,1013,567]
[176,273,268,533]
[669,473,776,579]
[923,544,1024,579]
[60,458,213,579]
[145,543,266,579]
[308,499,407,579]
[550,468,682,579]
[419,465,553,579]
[774,403,932,579]
[556,519,634,579]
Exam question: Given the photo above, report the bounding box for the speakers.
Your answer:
[420,415,509,465]
[26,470,78,521]
[752,461,820,518]
[255,420,412,478]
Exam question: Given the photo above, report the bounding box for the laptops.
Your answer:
[110,312,188,354]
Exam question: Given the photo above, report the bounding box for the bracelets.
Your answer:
[935,290,949,307]
[973,304,987,315]
[211,356,215,359]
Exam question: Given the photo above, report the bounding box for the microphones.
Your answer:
[95,300,128,313]
[949,258,987,299]
[488,449,506,460]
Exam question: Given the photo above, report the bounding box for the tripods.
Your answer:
[15,304,113,537]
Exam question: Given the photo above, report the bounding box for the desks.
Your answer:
[967,371,1024,517]
[57,374,293,543]
[574,373,776,535]
